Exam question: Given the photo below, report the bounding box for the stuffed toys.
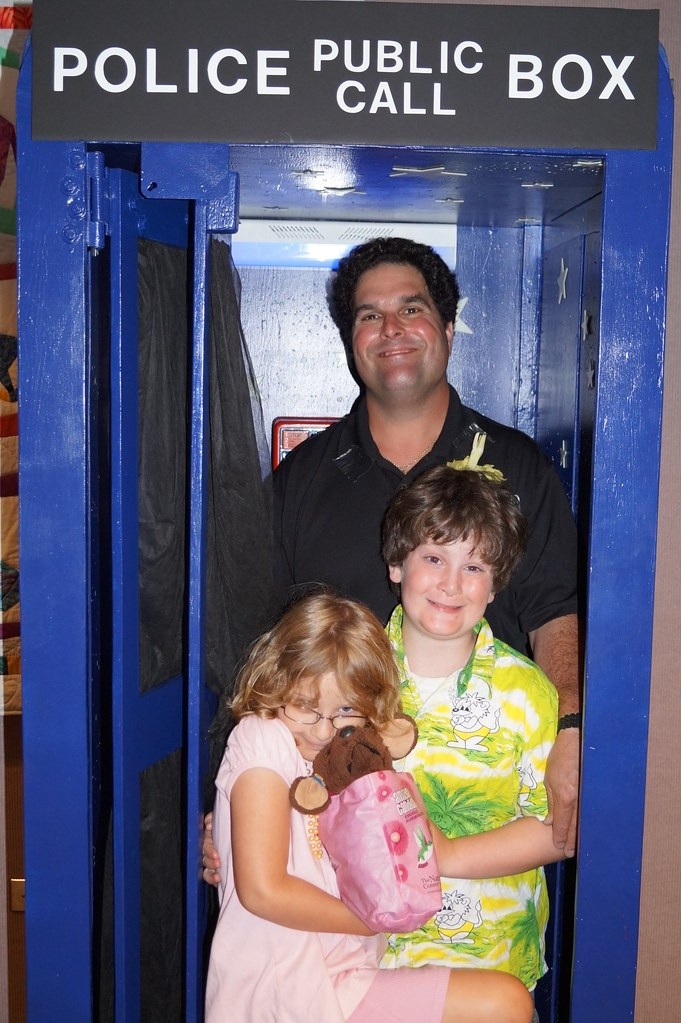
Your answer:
[288,711,418,815]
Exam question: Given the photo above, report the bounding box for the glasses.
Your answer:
[278,701,371,732]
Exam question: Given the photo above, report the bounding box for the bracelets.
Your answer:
[557,712,582,731]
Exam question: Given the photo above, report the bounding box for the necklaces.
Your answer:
[303,762,322,859]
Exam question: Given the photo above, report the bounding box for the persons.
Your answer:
[200,471,560,1023]
[273,235,583,857]
[205,596,533,1023]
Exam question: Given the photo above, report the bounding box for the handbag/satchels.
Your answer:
[317,769,442,934]
